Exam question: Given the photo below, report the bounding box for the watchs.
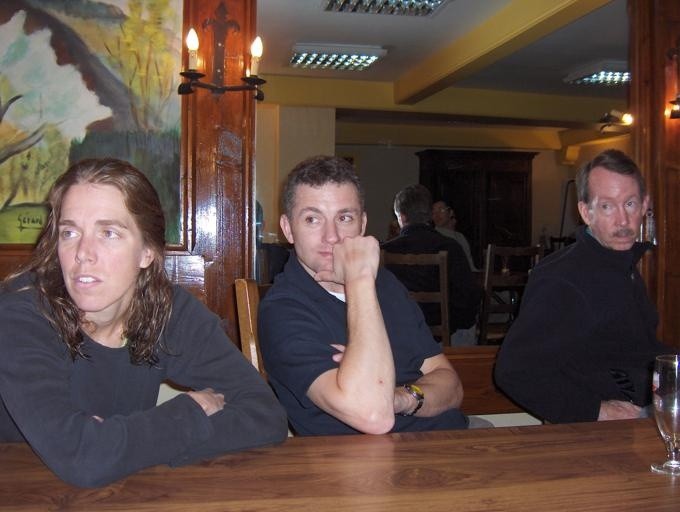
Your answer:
[402,383,424,417]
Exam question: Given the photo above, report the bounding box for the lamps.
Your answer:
[324,0,448,18]
[601,109,633,130]
[289,43,387,72]
[178,3,266,101]
[564,61,629,87]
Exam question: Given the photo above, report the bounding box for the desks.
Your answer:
[0,418,680,512]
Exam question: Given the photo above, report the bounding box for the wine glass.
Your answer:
[648,353,680,477]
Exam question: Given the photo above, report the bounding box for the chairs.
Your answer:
[235,279,272,382]
[381,250,451,346]
[478,244,544,345]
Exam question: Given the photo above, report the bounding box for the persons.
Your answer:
[494,147,678,426]
[255,157,494,436]
[0,158,288,489]
[377,185,483,345]
